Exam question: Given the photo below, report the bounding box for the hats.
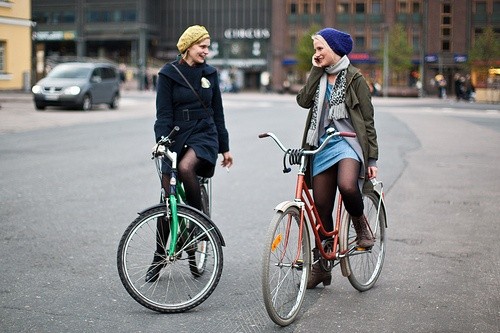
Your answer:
[316,27,353,56]
[176,25,210,55]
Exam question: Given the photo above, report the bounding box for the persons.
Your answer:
[297,28,378,291]
[119,62,290,96]
[366,72,476,103]
[144,25,233,283]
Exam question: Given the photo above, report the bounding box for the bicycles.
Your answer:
[258,132,389,327]
[117,126,226,313]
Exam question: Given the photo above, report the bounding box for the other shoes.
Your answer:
[145,251,168,285]
[191,225,209,240]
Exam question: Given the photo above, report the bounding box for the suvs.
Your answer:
[31,62,121,111]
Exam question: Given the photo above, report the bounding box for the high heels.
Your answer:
[355,217,375,248]
[296,248,332,288]
